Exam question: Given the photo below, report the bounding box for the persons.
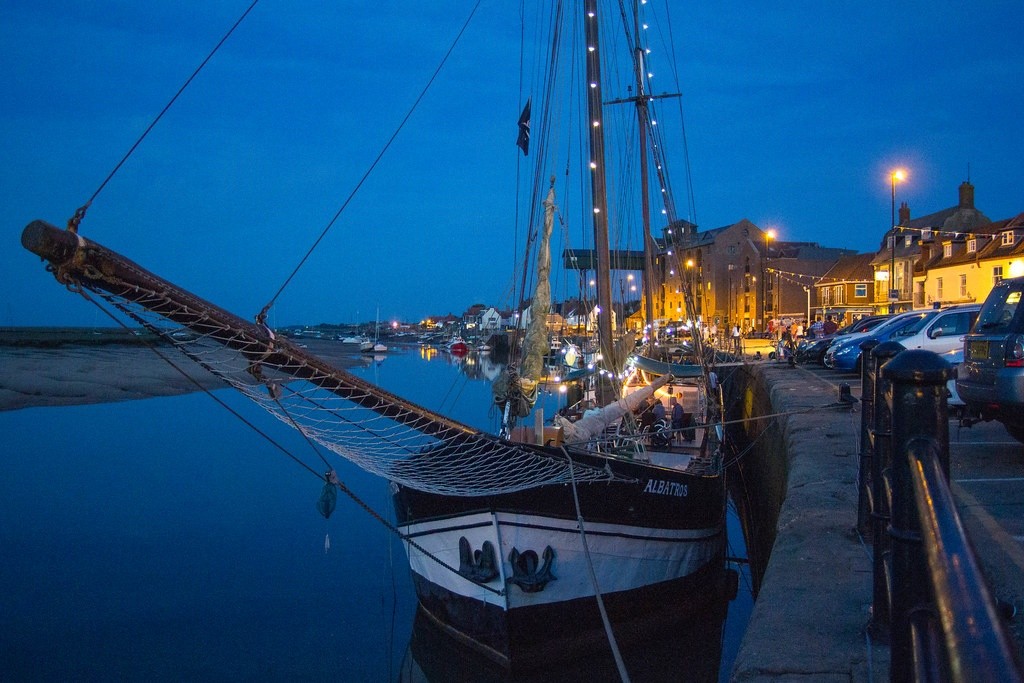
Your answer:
[731,323,741,348]
[767,315,839,339]
[671,398,684,429]
[639,397,666,418]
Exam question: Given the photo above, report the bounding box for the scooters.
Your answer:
[769,339,796,360]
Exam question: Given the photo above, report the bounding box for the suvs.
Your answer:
[956,277,1024,444]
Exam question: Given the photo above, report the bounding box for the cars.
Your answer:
[794,309,956,381]
[896,303,1018,406]
[663,321,691,338]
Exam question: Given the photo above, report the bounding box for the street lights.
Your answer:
[628,275,638,318]
[590,279,597,311]
[891,168,907,299]
[762,229,776,333]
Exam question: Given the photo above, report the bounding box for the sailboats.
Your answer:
[18,0,751,677]
